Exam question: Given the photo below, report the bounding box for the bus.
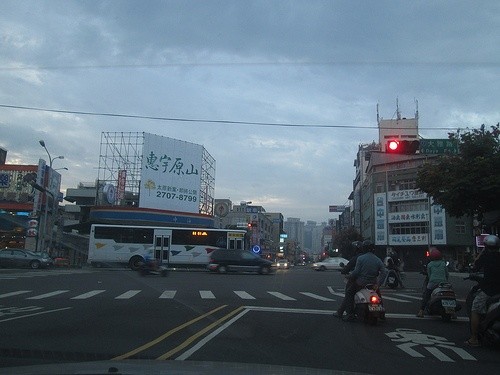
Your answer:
[88,224,248,270]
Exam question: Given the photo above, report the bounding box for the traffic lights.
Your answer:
[386,139,421,155]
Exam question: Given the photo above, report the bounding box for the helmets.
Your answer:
[362,240,373,251]
[430,247,440,258]
[351,241,362,249]
[482,234,500,247]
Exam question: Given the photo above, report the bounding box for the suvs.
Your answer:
[207,248,271,274]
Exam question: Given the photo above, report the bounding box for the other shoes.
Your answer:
[342,310,351,316]
[379,316,386,323]
[463,338,480,349]
[416,310,424,318]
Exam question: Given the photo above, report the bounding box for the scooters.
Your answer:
[464,274,500,348]
[340,262,384,325]
[420,265,457,324]
[386,262,401,288]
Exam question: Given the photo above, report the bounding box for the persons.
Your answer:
[332,239,406,322]
[461,234,500,347]
[417,247,449,317]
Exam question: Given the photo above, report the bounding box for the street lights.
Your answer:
[38,140,64,254]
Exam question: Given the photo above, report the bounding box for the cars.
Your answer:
[277,260,290,269]
[140,260,168,276]
[0,248,55,269]
[312,257,349,271]
